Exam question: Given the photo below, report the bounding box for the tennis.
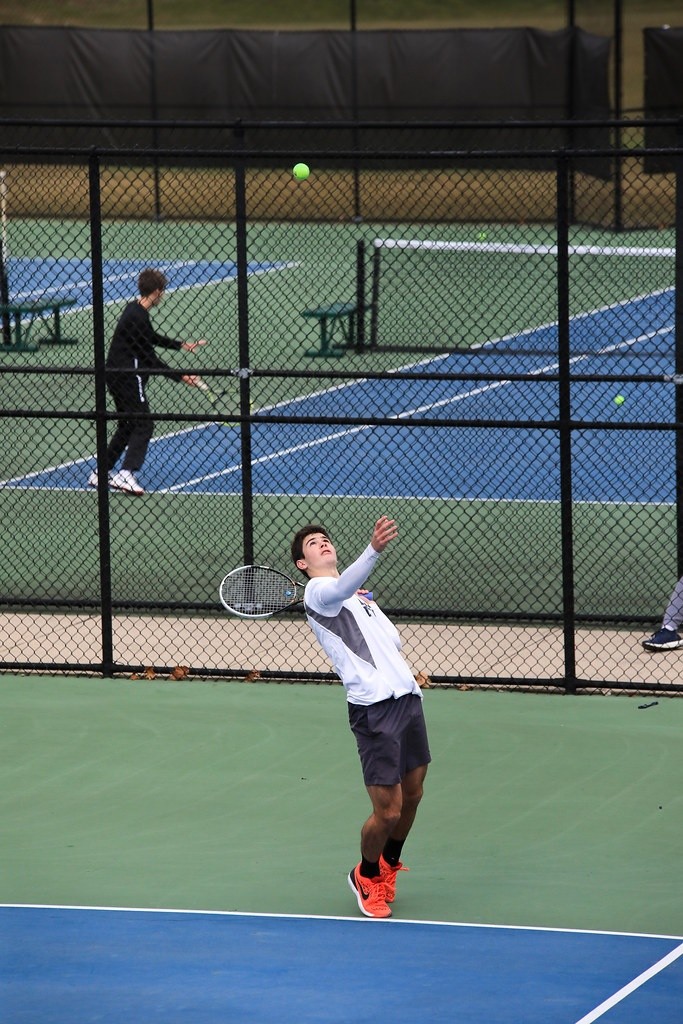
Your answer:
[478,233,487,242]
[293,163,310,180]
[614,395,624,404]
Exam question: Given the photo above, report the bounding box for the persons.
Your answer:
[641,577,683,651]
[290,515,433,919]
[88,270,208,494]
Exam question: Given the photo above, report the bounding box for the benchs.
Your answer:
[0,298,78,353]
[301,301,372,358]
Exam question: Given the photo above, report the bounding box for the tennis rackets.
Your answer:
[192,375,253,428]
[220,565,373,618]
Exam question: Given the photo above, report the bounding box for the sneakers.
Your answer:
[346,852,410,918]
[642,627,683,651]
[107,470,145,494]
[88,468,110,490]
